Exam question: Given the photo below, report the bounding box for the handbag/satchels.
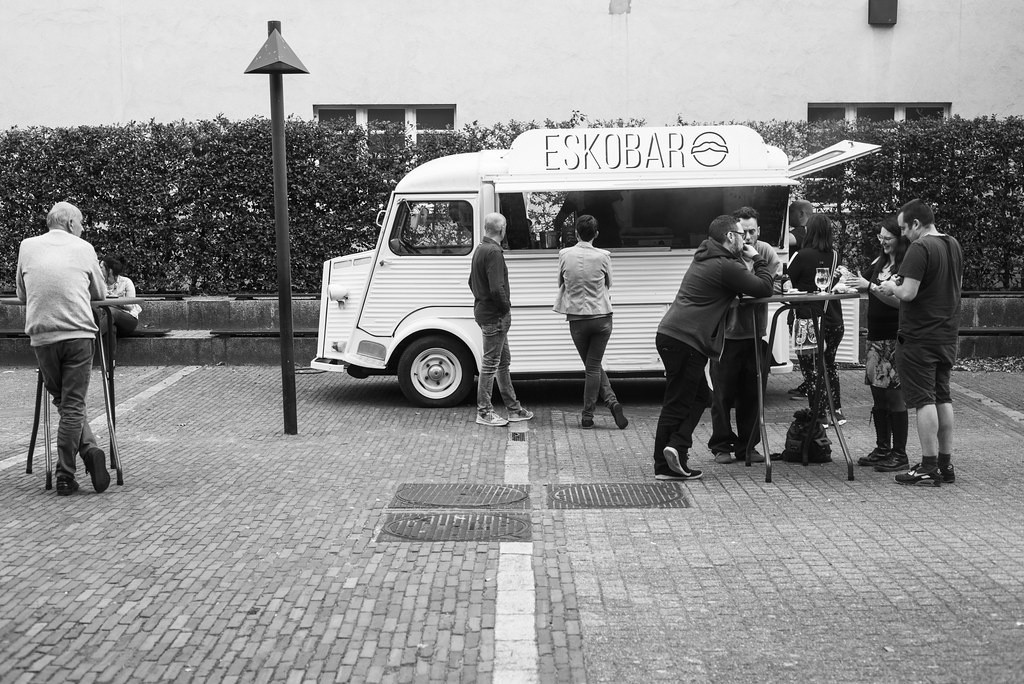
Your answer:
[790,318,828,355]
[780,409,834,463]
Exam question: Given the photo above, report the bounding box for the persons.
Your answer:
[708,208,784,464]
[16,202,111,494]
[100,257,142,371]
[788,201,845,428]
[553,216,628,428]
[833,220,912,472]
[468,212,534,425]
[654,215,773,480]
[879,199,964,486]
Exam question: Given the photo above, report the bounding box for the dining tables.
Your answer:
[1,298,148,489]
[734,294,863,482]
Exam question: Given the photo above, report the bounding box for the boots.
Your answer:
[858,408,909,472]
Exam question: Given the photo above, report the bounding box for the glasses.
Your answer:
[724,231,747,240]
[875,233,897,242]
[742,229,756,236]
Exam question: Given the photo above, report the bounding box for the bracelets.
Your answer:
[867,281,876,293]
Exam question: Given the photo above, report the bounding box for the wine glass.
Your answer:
[815,267,832,296]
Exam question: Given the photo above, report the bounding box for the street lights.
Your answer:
[244,20,310,434]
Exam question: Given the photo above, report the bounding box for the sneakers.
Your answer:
[893,462,943,487]
[828,407,847,426]
[83,447,110,494]
[475,411,510,427]
[911,461,956,483]
[818,415,830,429]
[662,445,692,477]
[507,406,534,422]
[56,475,80,496]
[654,464,704,480]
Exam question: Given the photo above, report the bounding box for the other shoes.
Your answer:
[581,417,595,429]
[740,448,766,462]
[787,382,810,400]
[610,401,629,429]
[714,450,733,463]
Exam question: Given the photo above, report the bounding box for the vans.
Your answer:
[311,125,883,407]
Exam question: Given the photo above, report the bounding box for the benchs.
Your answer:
[957,289,1024,362]
[0,293,319,366]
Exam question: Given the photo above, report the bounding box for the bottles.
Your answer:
[781,263,791,294]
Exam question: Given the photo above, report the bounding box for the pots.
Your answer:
[539,231,558,248]
